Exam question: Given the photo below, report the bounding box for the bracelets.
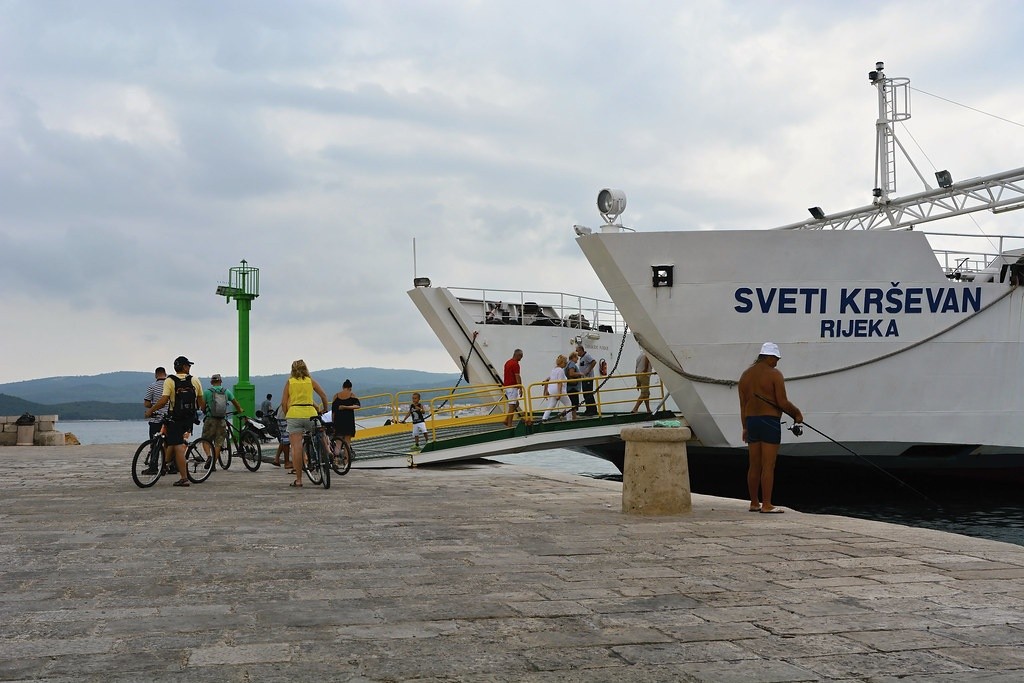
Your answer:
[420,409,423,411]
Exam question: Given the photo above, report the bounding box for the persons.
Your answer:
[401,393,431,450]
[738,342,803,514]
[631,352,653,414]
[542,355,576,422]
[142,357,361,487]
[576,345,597,416]
[504,348,524,427]
[564,352,583,418]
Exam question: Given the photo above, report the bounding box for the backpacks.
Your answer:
[261,404,283,438]
[167,374,198,424]
[208,387,229,417]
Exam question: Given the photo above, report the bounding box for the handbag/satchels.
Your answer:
[564,361,578,386]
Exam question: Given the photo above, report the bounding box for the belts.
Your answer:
[292,404,312,406]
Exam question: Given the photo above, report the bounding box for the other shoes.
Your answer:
[141,466,158,475]
[173,478,190,486]
[204,456,217,472]
[579,410,598,416]
[503,420,514,428]
[630,411,638,415]
[168,465,177,474]
[410,443,421,450]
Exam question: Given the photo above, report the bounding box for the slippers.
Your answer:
[290,480,303,487]
[748,504,762,511]
[328,451,334,465]
[760,507,784,513]
[284,462,293,468]
[272,460,281,467]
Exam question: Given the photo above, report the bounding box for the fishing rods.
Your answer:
[752,391,944,512]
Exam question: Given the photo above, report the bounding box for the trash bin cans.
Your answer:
[16,415,35,446]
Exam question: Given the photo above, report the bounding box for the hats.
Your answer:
[212,374,221,381]
[759,342,781,359]
[174,356,194,366]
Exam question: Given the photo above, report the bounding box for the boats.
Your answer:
[573,61,1024,455]
[407,232,683,419]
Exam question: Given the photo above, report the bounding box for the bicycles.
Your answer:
[132,412,214,488]
[217,410,264,472]
[323,422,354,475]
[303,410,335,491]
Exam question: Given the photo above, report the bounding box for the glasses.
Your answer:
[186,364,191,367]
